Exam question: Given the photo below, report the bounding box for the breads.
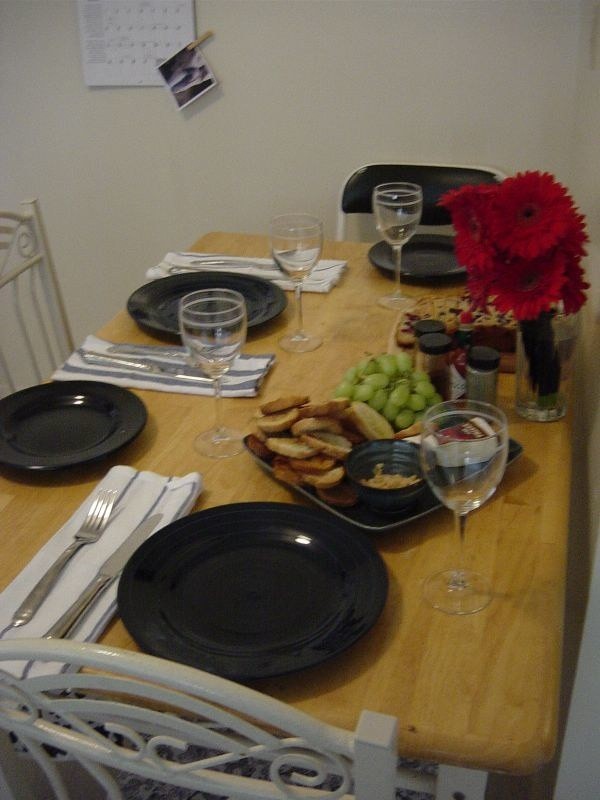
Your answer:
[247,394,438,506]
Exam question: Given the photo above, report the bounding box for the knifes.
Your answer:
[41,513,165,638]
[81,350,215,382]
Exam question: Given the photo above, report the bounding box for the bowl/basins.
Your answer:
[342,439,428,518]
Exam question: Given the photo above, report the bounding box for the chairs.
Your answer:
[0,637,399,800]
[332,164,508,244]
[0,198,76,402]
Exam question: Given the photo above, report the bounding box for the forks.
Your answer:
[12,488,117,628]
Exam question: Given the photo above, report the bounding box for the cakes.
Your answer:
[394,293,577,361]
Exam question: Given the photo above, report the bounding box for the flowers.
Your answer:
[435,168,591,321]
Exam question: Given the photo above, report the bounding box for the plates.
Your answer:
[368,234,465,283]
[0,381,148,470]
[126,271,288,335]
[240,432,524,531]
[118,502,390,677]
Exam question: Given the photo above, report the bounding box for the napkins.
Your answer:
[0,465,203,691]
[144,249,347,294]
[49,334,276,398]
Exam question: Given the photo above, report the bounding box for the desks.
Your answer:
[0,230,574,800]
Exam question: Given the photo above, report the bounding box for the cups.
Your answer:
[514,306,576,421]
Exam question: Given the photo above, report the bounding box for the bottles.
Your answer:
[415,312,499,422]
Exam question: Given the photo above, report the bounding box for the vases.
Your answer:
[513,308,580,421]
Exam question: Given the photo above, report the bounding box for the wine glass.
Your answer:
[179,287,248,459]
[420,399,509,615]
[374,182,422,311]
[269,213,323,353]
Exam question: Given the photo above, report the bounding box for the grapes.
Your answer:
[336,350,443,427]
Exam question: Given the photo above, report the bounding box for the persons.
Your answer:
[171,52,206,93]
